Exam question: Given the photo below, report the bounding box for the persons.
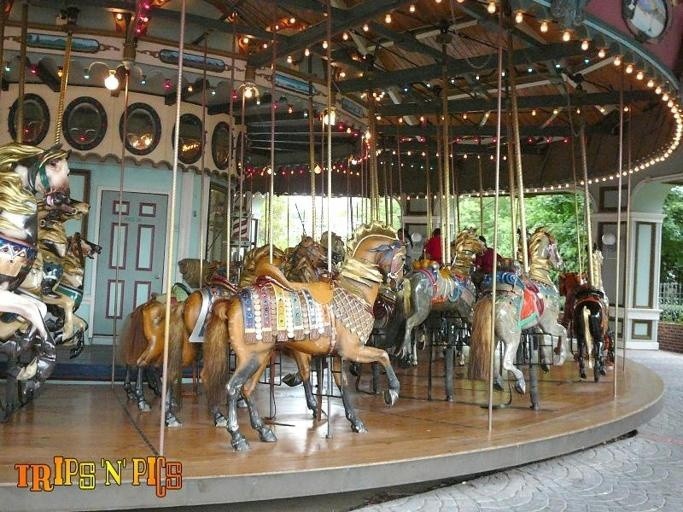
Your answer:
[0,143,613,449]
